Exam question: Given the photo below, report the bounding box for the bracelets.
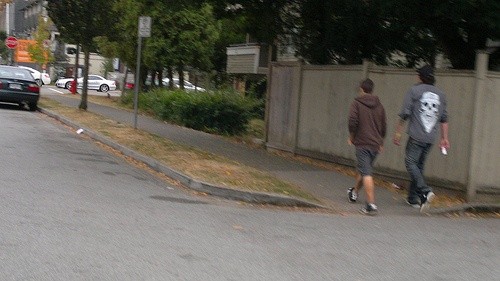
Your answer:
[395,133,401,139]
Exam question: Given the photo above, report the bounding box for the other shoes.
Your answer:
[405,198,421,208]
[418,190,435,214]
[359,202,378,215]
[347,186,358,203]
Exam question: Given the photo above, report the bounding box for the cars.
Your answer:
[55,74,116,93]
[0,66,51,111]
[125,75,206,94]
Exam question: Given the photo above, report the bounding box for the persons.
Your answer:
[347,78,386,216]
[393,65,449,215]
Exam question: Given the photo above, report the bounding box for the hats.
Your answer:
[415,64,433,74]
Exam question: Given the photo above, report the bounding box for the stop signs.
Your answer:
[5,36,19,49]
